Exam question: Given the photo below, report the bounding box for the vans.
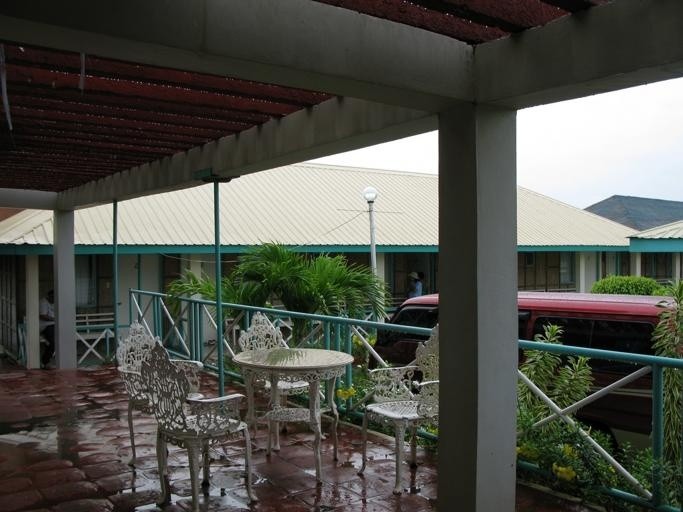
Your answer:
[373,290,678,454]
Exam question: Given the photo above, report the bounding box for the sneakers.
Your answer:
[40,362,55,370]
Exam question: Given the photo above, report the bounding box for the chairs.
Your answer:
[140,335,260,512]
[237,310,327,457]
[356,323,439,476]
[115,319,205,468]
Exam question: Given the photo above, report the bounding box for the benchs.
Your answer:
[19,312,115,369]
[225,297,407,345]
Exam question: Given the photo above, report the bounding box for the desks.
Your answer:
[231,347,355,487]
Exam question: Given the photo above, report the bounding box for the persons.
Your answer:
[38,289,55,370]
[405,271,423,299]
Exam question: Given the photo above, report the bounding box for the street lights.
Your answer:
[361,185,381,320]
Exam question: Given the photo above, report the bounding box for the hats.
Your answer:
[406,270,421,280]
